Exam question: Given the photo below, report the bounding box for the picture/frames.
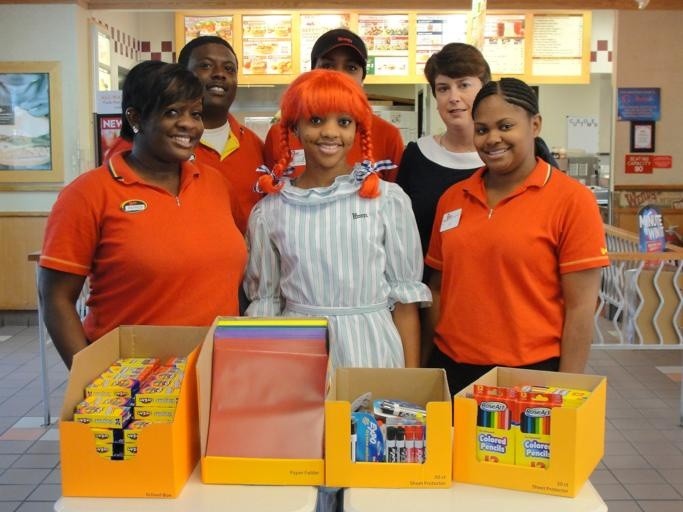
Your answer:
[0,61,64,182]
[630,120,656,152]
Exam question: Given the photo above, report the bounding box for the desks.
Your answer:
[342,477,611,511]
[54,461,319,512]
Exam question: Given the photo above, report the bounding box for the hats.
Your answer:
[310,28,369,68]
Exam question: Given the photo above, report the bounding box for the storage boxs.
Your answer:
[454,366,606,498]
[195,316,332,486]
[325,367,453,488]
[58,325,209,499]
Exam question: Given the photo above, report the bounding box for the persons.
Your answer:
[424,78,611,427]
[37,60,249,371]
[103,36,266,316]
[395,43,559,368]
[264,28,404,183]
[240,69,433,512]
